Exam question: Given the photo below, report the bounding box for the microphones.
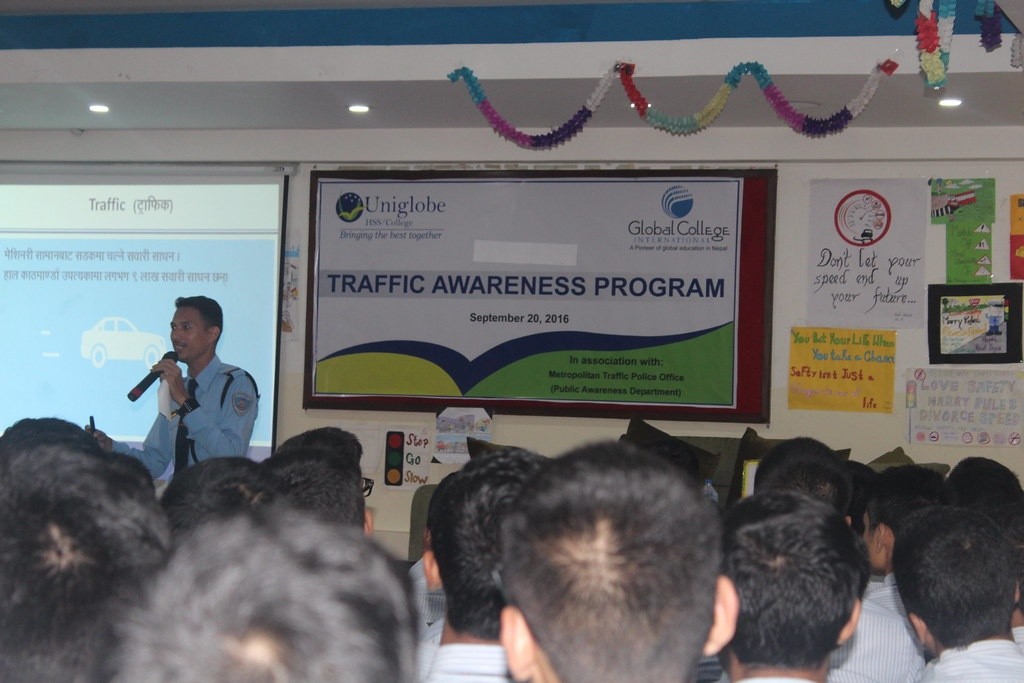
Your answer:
[128,351,179,402]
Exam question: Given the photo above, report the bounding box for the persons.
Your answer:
[84,293,260,502]
[0,417,1024,683]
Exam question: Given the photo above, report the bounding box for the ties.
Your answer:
[175,379,197,471]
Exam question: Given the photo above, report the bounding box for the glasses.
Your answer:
[362,478,374,497]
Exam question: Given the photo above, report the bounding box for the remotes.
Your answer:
[90,416,95,434]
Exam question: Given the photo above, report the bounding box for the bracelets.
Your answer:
[175,397,200,418]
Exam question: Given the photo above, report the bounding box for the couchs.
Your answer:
[674,435,792,514]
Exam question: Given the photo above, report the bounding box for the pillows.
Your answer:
[727,424,852,510]
[617,414,721,489]
[866,446,953,479]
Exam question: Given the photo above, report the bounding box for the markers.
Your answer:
[88,414,97,436]
